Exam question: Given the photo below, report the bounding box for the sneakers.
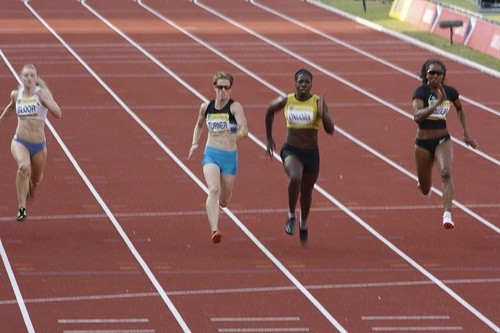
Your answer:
[16,207,26,221]
[443,211,454,228]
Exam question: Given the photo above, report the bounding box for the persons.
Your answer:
[265,69,335,244]
[0,63,62,221]
[188,71,248,243]
[413,60,478,229]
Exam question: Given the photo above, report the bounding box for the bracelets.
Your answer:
[193,143,198,145]
[239,131,244,139]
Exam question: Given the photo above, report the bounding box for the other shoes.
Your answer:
[212,231,222,243]
[299,221,308,241]
[284,216,296,234]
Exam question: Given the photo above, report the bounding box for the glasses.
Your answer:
[214,83,232,90]
[427,70,444,76]
